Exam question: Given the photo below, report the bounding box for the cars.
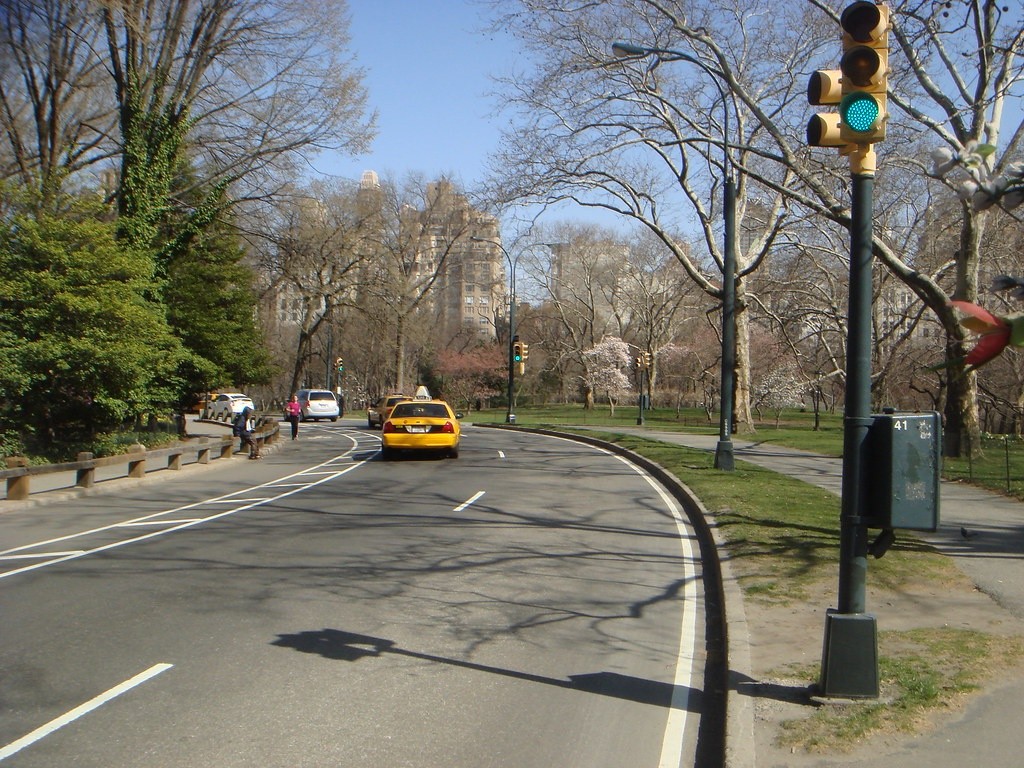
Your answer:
[188,393,255,421]
[281,389,340,423]
[366,385,465,459]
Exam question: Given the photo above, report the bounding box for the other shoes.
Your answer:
[250,455,260,459]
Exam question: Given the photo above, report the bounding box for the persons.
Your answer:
[234,406,260,459]
[285,393,301,443]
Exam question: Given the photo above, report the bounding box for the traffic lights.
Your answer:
[333,356,343,373]
[636,351,651,370]
[513,342,530,364]
[805,0,888,149]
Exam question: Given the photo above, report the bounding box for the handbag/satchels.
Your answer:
[283,409,291,422]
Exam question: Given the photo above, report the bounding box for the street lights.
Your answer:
[470,235,563,428]
[610,42,737,471]
[309,289,332,389]
